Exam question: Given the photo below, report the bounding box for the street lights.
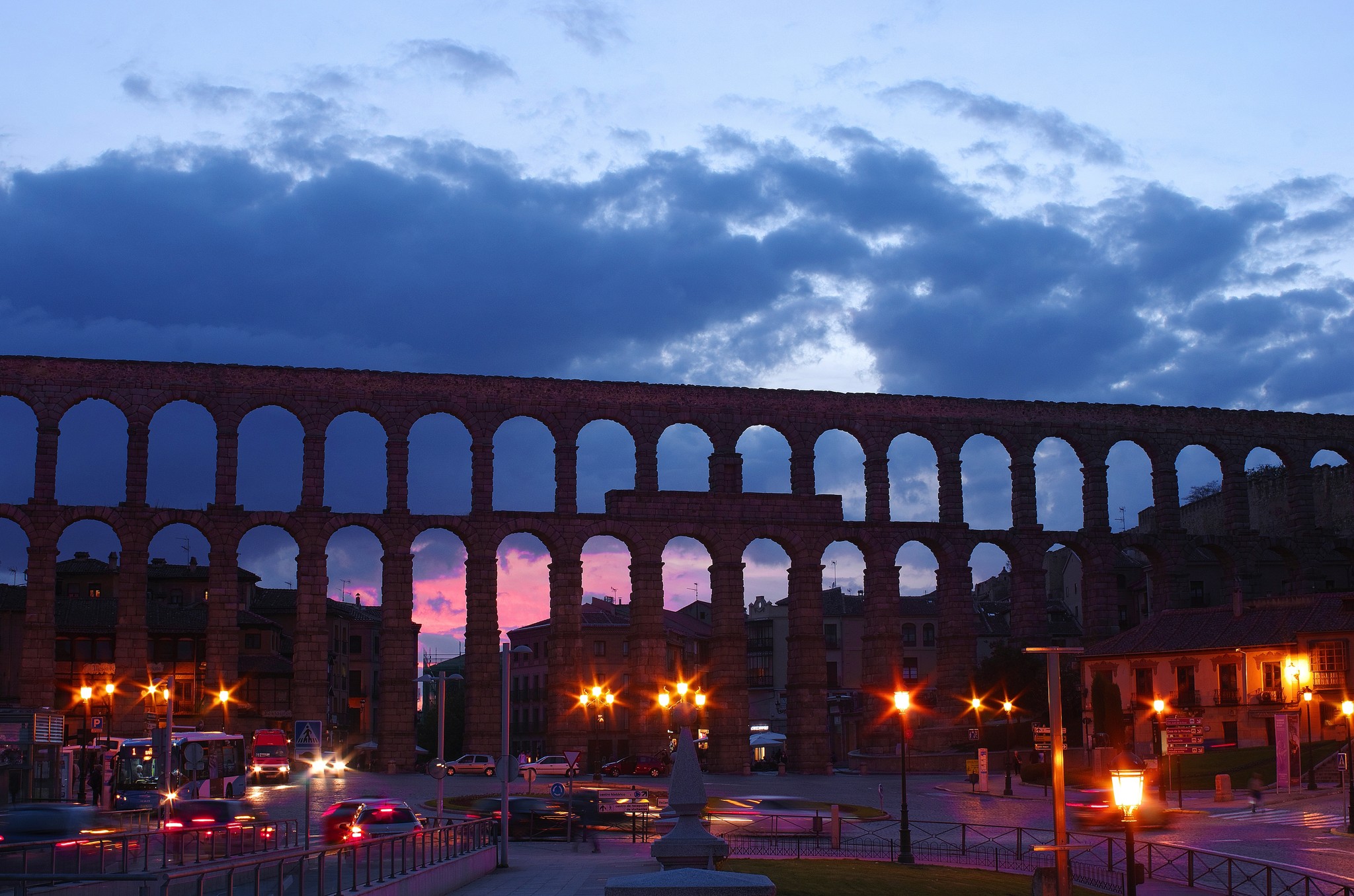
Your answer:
[1154,699,1166,801]
[892,681,916,863]
[411,671,465,831]
[500,643,533,868]
[1003,698,1013,795]
[972,695,980,760]
[77,676,229,830]
[580,685,614,784]
[1108,743,1148,896]
[1303,686,1318,790]
[1341,700,1354,834]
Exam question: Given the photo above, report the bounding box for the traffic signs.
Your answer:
[1034,727,1067,751]
[1166,717,1204,754]
[597,802,649,812]
[598,789,649,799]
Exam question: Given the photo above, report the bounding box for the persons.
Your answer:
[1012,751,1021,776]
[1,744,20,794]
[1029,749,1044,764]
[136,765,143,779]
[518,749,531,765]
[355,748,378,772]
[602,756,606,765]
[71,753,103,807]
[608,754,615,763]
[275,746,284,757]
[661,752,672,777]
[830,752,837,762]
[754,749,788,770]
[467,756,473,762]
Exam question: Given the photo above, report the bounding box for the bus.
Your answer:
[109,732,247,817]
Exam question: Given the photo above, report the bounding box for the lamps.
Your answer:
[347,698,365,714]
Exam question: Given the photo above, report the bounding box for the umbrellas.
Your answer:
[749,732,786,751]
[354,741,379,772]
[415,746,429,755]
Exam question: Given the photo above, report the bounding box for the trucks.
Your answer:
[247,728,292,785]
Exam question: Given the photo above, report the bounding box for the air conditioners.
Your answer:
[1261,691,1277,701]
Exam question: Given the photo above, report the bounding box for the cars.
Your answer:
[311,752,345,778]
[320,797,843,851]
[1063,788,1170,829]
[0,800,274,882]
[520,756,580,778]
[446,754,497,777]
[601,749,667,778]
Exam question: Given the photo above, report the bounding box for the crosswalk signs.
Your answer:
[1338,753,1347,771]
[294,720,322,748]
